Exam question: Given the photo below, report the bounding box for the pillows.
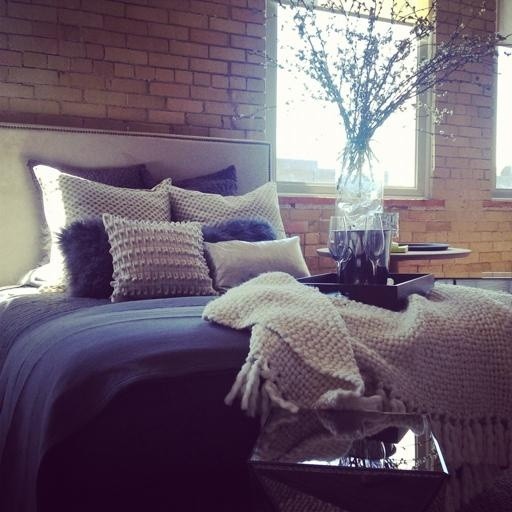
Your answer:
[200,217,280,294]
[28,160,158,192]
[201,234,310,294]
[32,164,174,294]
[53,217,114,300]
[169,164,237,198]
[170,179,287,243]
[102,212,220,303]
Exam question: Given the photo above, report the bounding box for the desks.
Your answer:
[317,243,473,274]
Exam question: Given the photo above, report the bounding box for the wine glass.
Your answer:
[328,217,352,283]
[365,216,386,283]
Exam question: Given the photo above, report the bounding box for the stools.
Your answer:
[247,406,449,511]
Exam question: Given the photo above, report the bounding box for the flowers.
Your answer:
[231,0,512,199]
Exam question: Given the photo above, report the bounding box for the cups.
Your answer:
[379,212,400,244]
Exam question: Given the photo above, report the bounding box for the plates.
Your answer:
[397,243,451,251]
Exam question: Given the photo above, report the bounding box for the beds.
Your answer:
[1,121,512,511]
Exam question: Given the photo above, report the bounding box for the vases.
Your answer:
[334,138,382,232]
